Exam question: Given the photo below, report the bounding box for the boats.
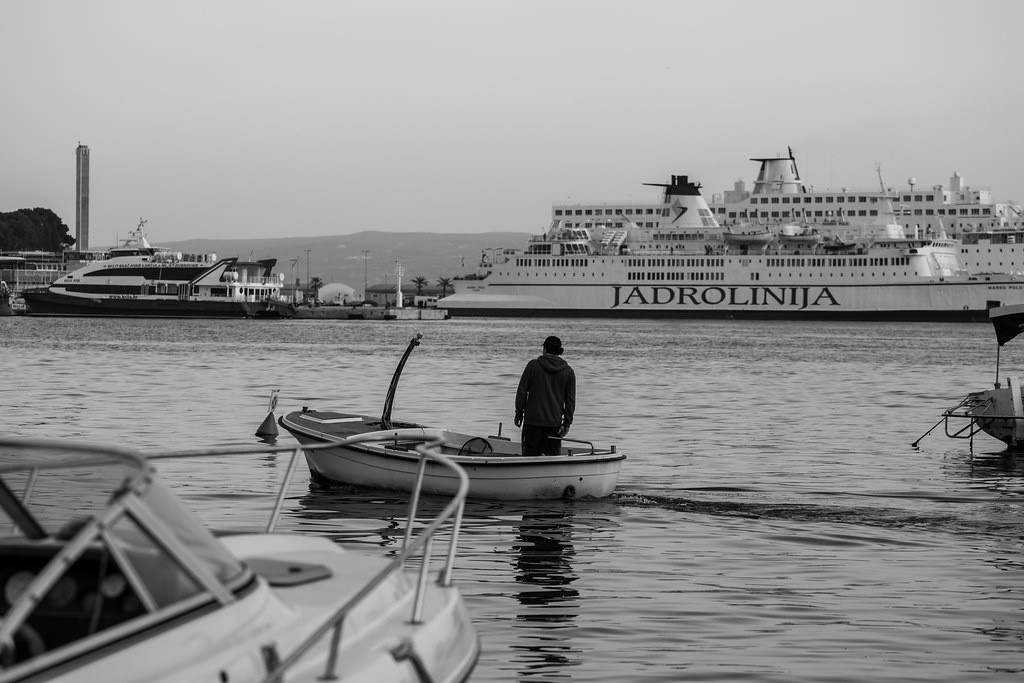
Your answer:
[778,224,822,251]
[722,230,774,246]
[276,299,386,321]
[823,240,856,251]
[939,302,1024,452]
[1,416,482,683]
[19,216,286,321]
[274,330,627,504]
[0,280,32,317]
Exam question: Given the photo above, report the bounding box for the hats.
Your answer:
[541,336,562,347]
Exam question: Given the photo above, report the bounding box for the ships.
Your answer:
[433,146,1024,325]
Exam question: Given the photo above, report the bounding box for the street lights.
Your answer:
[304,248,312,305]
[361,250,370,304]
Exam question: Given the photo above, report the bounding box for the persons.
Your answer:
[704,245,712,255]
[514,336,576,456]
[927,224,932,234]
